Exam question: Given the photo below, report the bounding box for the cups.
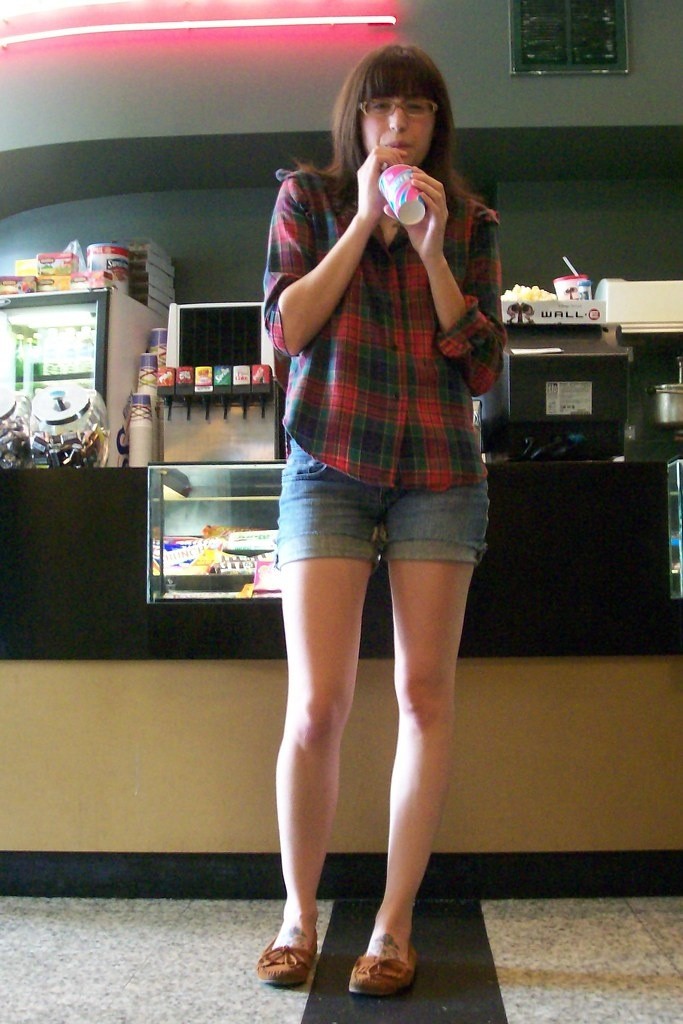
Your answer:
[378,163,426,226]
[129,327,168,468]
[554,274,589,301]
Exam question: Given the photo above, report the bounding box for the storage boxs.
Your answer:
[122,237,176,316]
[0,253,115,296]
[501,299,607,324]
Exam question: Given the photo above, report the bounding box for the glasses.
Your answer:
[358,99,438,118]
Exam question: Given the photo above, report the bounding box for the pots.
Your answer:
[647,383,683,428]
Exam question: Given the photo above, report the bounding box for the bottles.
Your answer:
[579,280,592,301]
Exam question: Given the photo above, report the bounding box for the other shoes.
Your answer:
[349,943,418,995]
[256,934,317,985]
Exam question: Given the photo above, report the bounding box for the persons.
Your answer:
[255,46,508,994]
